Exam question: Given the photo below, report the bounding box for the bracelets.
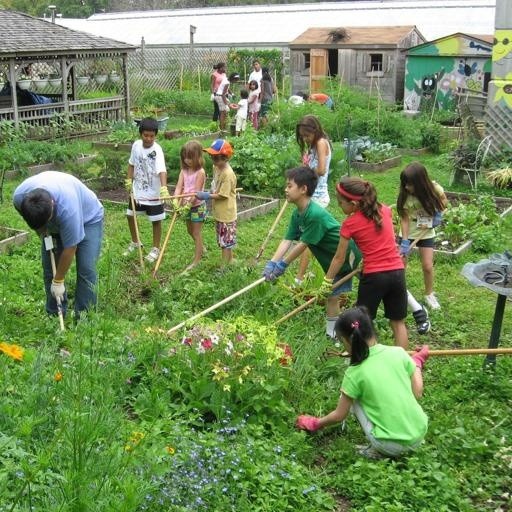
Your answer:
[53,278,65,283]
[324,276,333,283]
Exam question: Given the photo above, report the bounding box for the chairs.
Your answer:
[447,133,495,193]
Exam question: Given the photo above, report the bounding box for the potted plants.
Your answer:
[0,56,122,93]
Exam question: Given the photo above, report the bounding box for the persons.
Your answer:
[294,305,430,460]
[172,140,210,273]
[195,139,238,274]
[296,115,332,208]
[262,166,430,348]
[210,60,276,139]
[13,171,104,324]
[121,117,172,264]
[302,93,334,108]
[395,161,448,310]
[317,177,408,349]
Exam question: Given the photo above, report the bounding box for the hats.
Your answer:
[201,139,233,160]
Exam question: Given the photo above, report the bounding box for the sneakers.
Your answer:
[412,303,431,335]
[425,292,441,310]
[121,240,144,257]
[143,246,161,264]
[325,333,340,348]
[353,444,387,462]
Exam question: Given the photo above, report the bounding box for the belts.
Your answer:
[214,93,227,97]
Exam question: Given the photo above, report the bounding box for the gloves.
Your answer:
[410,344,430,371]
[399,239,411,259]
[432,210,443,229]
[48,277,66,302]
[193,191,210,200]
[171,198,194,218]
[317,275,335,301]
[356,257,364,274]
[123,178,133,195]
[294,414,322,432]
[263,260,277,282]
[159,186,173,207]
[270,259,289,281]
